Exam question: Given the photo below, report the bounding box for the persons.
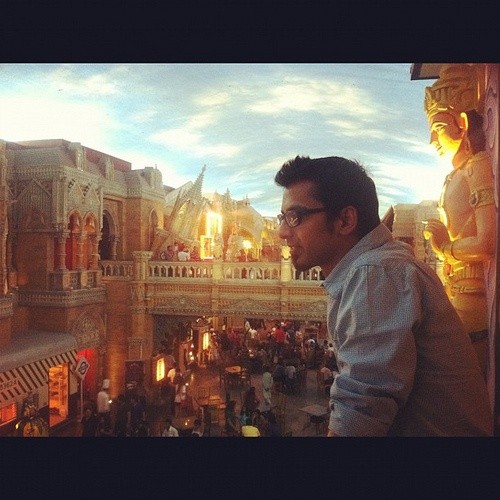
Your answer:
[77,234,335,437]
[420,64,496,332]
[274,154,494,436]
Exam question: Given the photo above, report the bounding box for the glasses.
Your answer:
[276,207,332,227]
